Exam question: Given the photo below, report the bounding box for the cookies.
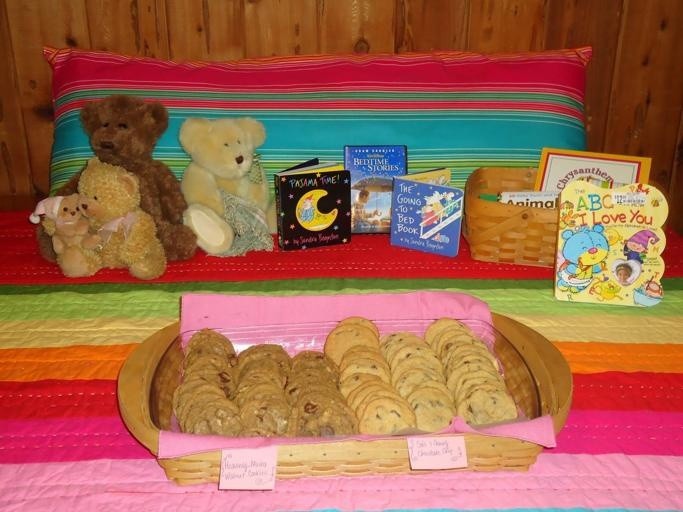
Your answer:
[171,316,519,440]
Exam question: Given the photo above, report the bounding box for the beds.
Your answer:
[2,206,682,508]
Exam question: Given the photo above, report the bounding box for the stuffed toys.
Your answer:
[34,92,196,267]
[177,116,279,256]
[28,192,100,252]
[56,156,167,281]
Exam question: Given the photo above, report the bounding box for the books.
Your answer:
[390,167,466,257]
[552,179,668,306]
[273,156,352,250]
[344,144,408,234]
[479,147,653,208]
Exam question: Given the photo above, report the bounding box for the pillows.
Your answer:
[40,44,595,225]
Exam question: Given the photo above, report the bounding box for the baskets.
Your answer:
[463,166,559,269]
[117,312,573,487]
